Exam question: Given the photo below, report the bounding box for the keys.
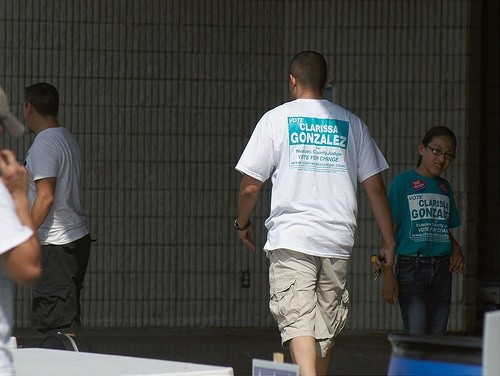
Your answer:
[370,255,388,281]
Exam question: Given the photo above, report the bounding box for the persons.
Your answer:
[233,50,398,376]
[387,126,467,335]
[0,85,44,376]
[21,81,93,334]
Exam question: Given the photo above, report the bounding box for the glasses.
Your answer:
[426,144,455,160]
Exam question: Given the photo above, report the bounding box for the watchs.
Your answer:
[233,216,251,231]
